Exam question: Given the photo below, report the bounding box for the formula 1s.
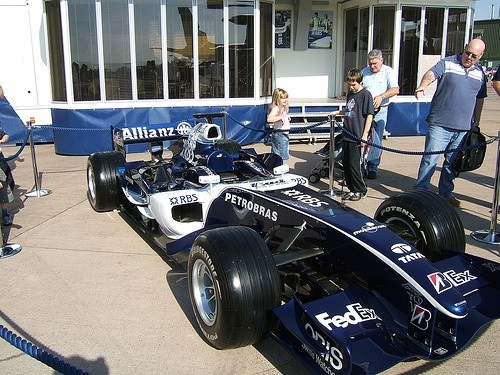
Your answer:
[86,113,500,374]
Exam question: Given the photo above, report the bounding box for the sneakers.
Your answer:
[448,196,461,207]
[2,213,12,225]
[368,172,376,179]
[341,192,366,201]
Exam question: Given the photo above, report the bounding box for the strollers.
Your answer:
[309,102,381,186]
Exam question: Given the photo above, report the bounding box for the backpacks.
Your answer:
[451,126,487,178]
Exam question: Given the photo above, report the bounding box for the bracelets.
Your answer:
[381,94,384,100]
[473,120,479,124]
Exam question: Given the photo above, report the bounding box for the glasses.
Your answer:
[465,50,478,59]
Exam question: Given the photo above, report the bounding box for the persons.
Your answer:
[310,12,332,32]
[414,38,500,206]
[147,59,156,70]
[267,88,291,160]
[72,61,92,100]
[427,38,435,55]
[0,127,15,226]
[328,49,400,201]
